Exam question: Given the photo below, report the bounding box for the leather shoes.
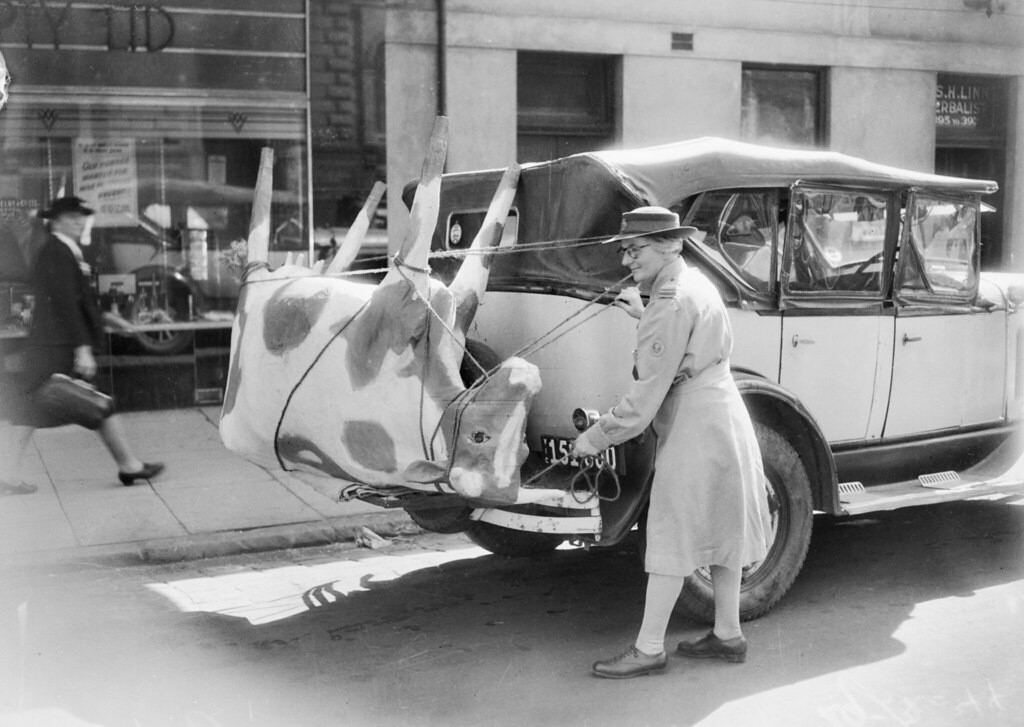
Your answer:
[676,631,747,663]
[592,644,668,679]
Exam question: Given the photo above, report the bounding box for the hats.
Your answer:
[37,197,94,219]
[602,206,698,244]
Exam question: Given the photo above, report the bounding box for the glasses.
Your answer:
[616,243,652,260]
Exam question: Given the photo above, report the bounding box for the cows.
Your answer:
[218,114,542,506]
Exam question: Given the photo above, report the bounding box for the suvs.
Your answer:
[67,178,306,356]
[401,139,1022,628]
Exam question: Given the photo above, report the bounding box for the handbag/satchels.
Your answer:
[36,369,113,431]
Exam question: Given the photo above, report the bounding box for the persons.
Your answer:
[571,205,773,678]
[0,198,165,496]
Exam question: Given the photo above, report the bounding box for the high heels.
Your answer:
[119,464,164,486]
[1,479,36,494]
